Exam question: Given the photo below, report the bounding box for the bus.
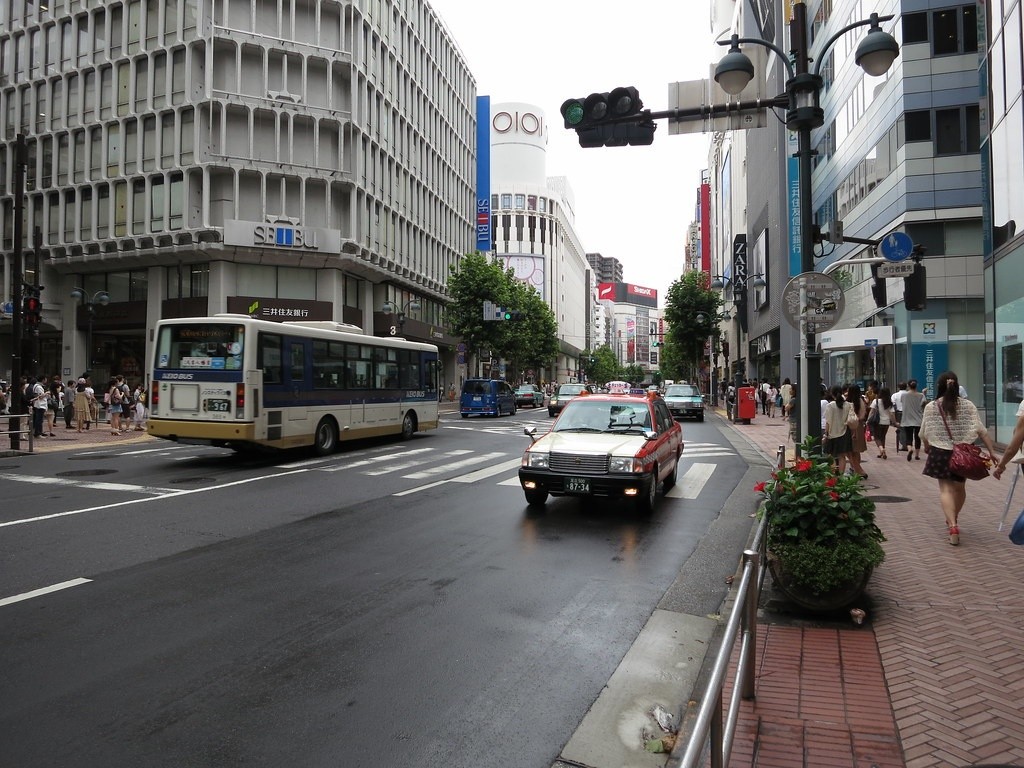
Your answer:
[144,313,444,458]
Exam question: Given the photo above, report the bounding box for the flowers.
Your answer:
[749,432,886,599]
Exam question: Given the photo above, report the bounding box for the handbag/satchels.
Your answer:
[868,399,880,426]
[949,443,993,480]
[47,398,58,410]
[758,392,766,399]
[104,393,111,404]
[111,388,123,406]
[766,399,771,404]
[848,403,859,430]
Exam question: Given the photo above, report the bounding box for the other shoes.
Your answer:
[877,453,883,458]
[899,448,908,451]
[915,456,920,460]
[849,468,854,472]
[19,423,145,440]
[907,450,913,461]
[860,474,868,479]
[882,450,887,459]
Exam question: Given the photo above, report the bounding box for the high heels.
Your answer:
[946,519,957,526]
[948,526,960,545]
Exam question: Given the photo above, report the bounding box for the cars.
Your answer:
[660,384,706,423]
[518,388,684,516]
[547,383,594,417]
[587,381,664,424]
[509,384,545,409]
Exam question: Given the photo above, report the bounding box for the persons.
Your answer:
[720,378,798,443]
[918,370,1001,545]
[104,375,149,434]
[532,381,557,405]
[818,376,968,479]
[0,372,98,439]
[448,379,457,402]
[993,399,1024,545]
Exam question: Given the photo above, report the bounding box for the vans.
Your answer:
[459,377,518,419]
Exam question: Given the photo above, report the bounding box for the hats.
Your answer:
[78,378,86,384]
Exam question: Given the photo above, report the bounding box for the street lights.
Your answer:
[711,272,767,420]
[69,285,112,389]
[382,299,422,338]
[696,310,732,408]
[711,11,900,453]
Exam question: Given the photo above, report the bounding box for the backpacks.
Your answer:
[116,383,126,401]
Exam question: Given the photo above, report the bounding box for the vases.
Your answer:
[767,522,873,610]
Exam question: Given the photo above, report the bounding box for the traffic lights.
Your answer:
[561,86,644,129]
[23,296,41,328]
[652,342,664,347]
[591,358,595,365]
[504,312,525,321]
